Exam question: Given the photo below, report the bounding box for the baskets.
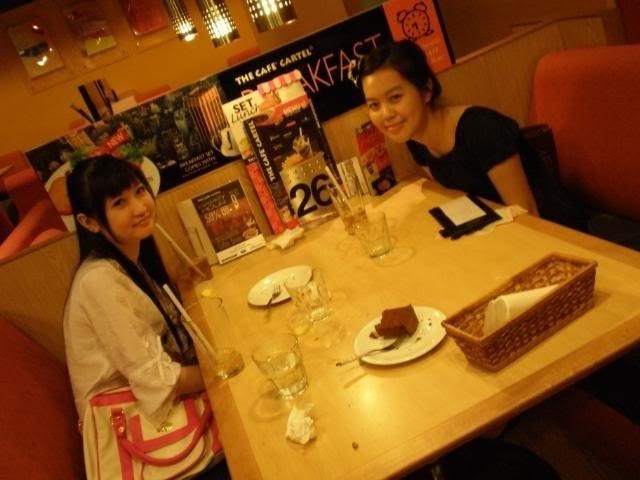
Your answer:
[441,252,598,373]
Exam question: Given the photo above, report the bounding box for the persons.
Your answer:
[61,154,234,480]
[358,38,561,221]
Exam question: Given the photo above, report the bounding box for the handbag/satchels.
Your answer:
[83,385,225,480]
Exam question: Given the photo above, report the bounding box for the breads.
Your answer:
[375,304,419,336]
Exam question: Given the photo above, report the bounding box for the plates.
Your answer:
[249,265,311,305]
[355,307,446,365]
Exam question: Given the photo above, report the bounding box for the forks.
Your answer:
[262,283,283,313]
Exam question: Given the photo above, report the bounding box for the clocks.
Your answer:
[396,0,435,42]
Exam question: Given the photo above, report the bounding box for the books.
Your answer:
[243,93,346,229]
[220,79,309,235]
[192,179,267,265]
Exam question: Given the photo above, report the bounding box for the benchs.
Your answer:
[0,317,85,479]
[529,44,640,424]
[0,47,262,260]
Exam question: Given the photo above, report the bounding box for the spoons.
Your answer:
[336,336,404,369]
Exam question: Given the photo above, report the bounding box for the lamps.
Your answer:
[163,0,298,48]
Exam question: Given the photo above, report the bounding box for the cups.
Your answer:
[251,334,310,400]
[326,185,393,258]
[181,296,244,380]
[288,270,333,327]
[171,229,213,280]
